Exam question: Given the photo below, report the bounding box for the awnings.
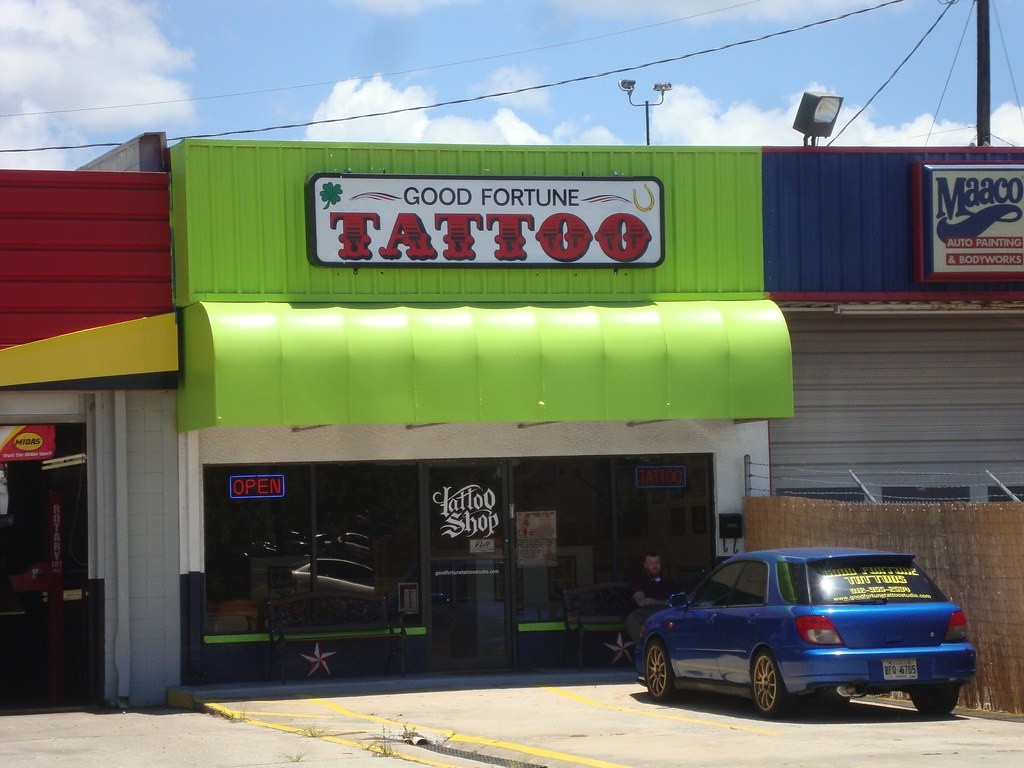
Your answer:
[180,297,796,437]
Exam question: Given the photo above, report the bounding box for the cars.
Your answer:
[633,545,979,721]
[239,519,474,637]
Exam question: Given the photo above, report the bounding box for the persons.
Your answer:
[633,550,680,629]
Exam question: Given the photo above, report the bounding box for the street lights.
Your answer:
[615,72,673,144]
